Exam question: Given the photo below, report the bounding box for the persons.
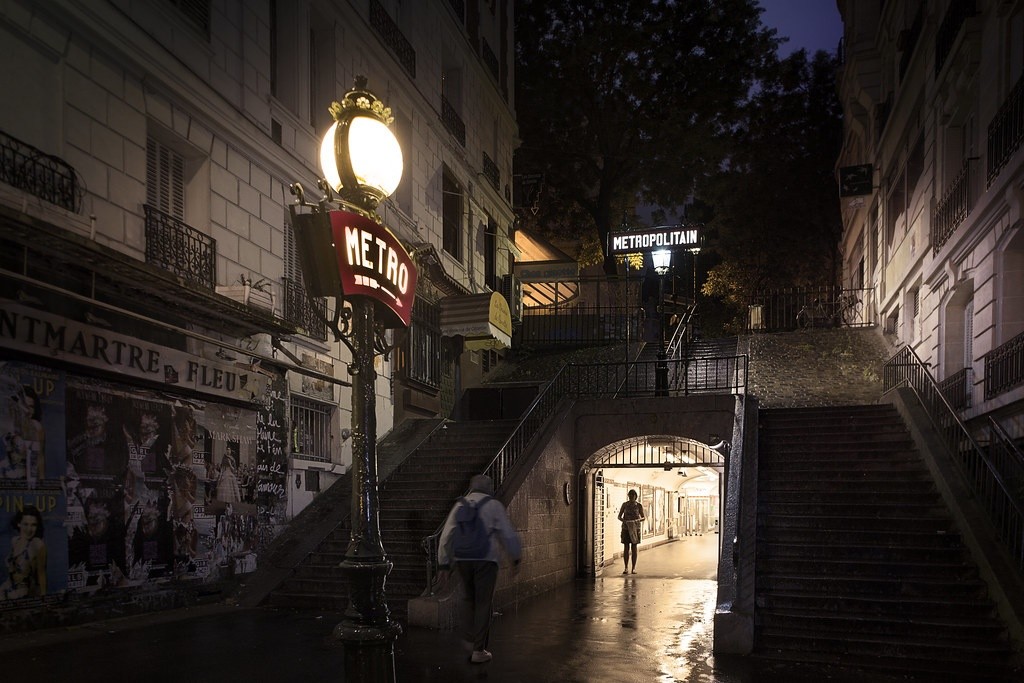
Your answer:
[617,489,647,573]
[433,473,522,661]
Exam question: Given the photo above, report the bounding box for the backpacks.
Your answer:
[451,496,498,558]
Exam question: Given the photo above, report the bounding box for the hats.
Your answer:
[470,474,494,495]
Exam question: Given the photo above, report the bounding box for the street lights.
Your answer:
[284,73,409,647]
[649,247,672,398]
[680,222,701,341]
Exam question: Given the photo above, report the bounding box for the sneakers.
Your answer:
[472,650,492,663]
[464,640,475,651]
